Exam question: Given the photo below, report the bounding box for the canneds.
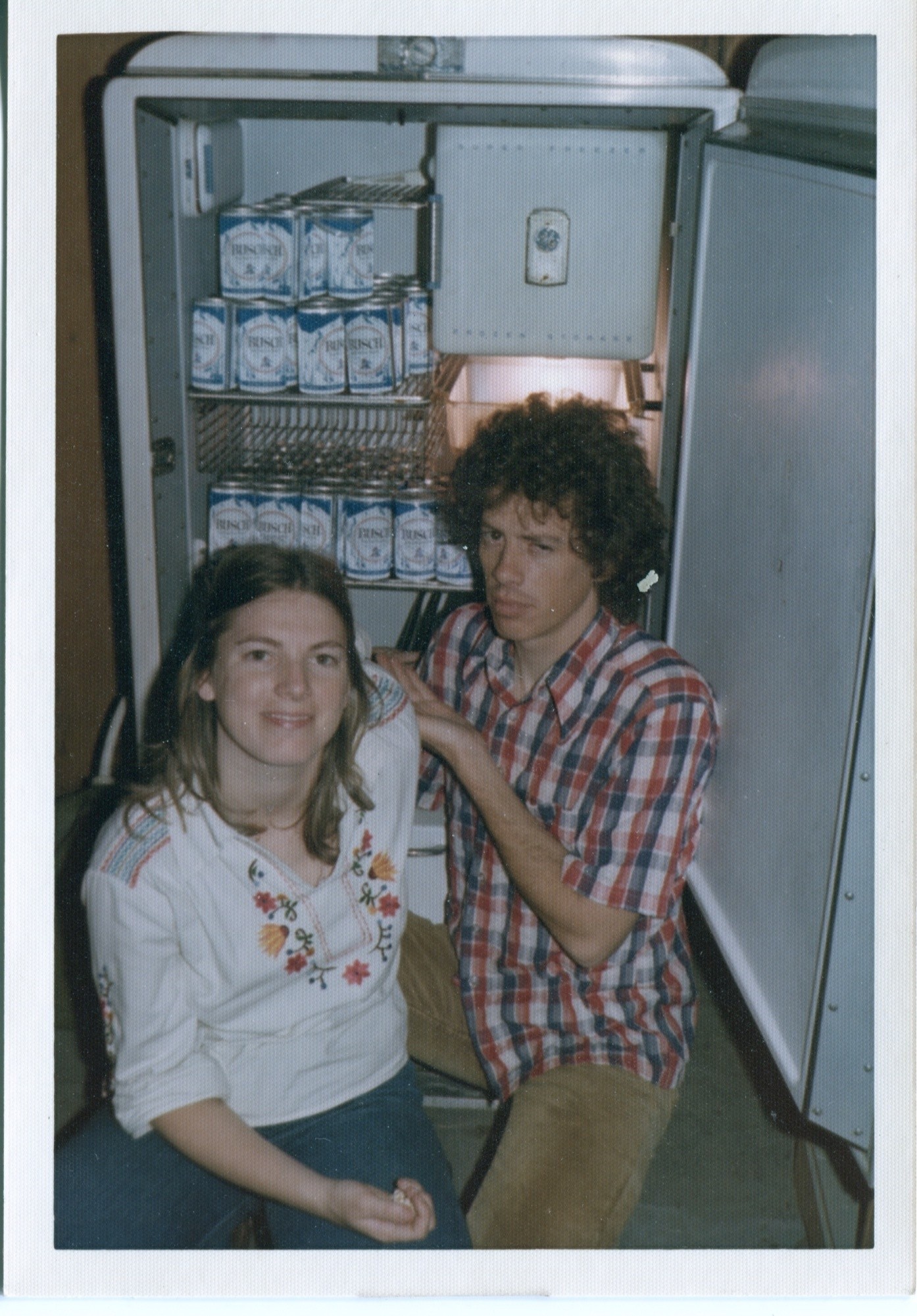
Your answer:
[219,191,376,302]
[208,440,475,585]
[191,272,439,395]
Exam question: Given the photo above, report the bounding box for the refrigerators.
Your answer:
[101,32,875,1154]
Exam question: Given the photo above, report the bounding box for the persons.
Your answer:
[54,538,471,1249]
[372,388,727,1249]
